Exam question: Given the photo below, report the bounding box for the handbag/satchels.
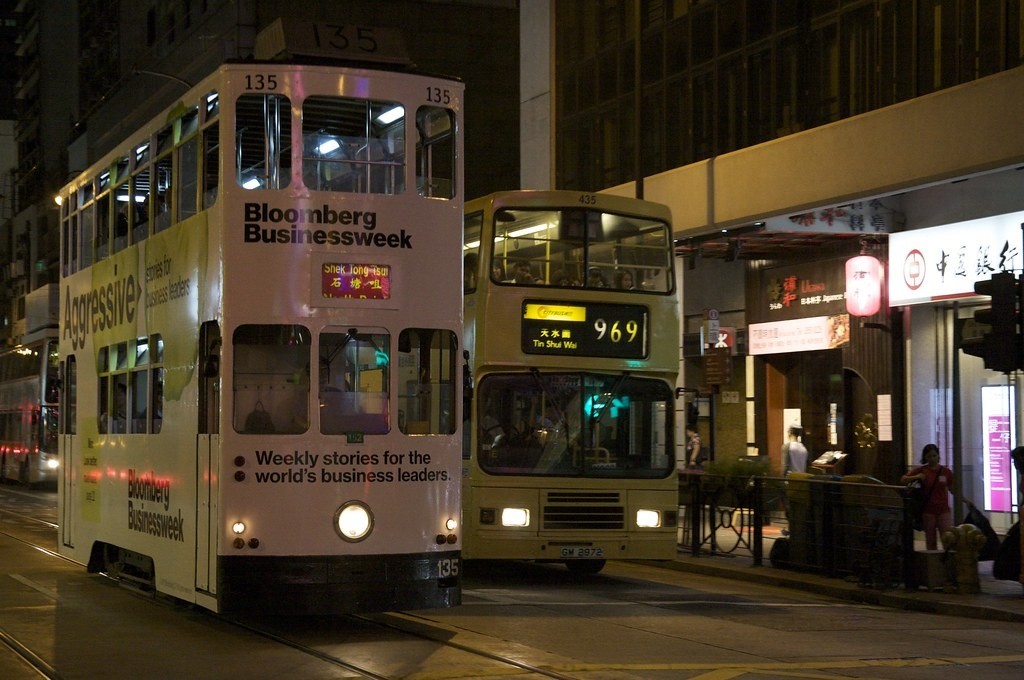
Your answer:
[992,521,1021,582]
[963,508,1003,561]
[911,511,922,530]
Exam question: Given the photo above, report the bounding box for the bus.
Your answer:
[342,188,700,582]
[46,64,476,615]
[0,282,62,489]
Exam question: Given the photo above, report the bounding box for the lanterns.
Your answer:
[845,255,881,323]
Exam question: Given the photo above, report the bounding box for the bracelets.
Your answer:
[690,459,695,462]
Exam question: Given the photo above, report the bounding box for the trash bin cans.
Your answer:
[769,471,904,588]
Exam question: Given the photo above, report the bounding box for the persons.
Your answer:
[685,424,702,469]
[101,383,140,433]
[901,444,954,550]
[102,188,185,258]
[464,257,633,292]
[294,356,352,433]
[780,424,808,474]
[137,381,163,434]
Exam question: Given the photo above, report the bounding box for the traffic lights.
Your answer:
[961,273,1017,375]
